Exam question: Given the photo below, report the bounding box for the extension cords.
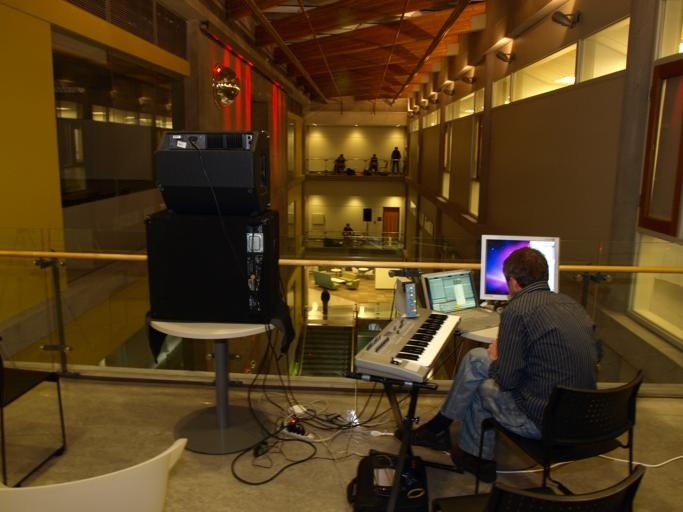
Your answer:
[277,421,313,441]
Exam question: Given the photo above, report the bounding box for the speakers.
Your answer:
[153,128,271,210]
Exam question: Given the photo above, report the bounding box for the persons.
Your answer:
[319,285,330,317]
[340,221,353,237]
[367,153,377,173]
[393,246,603,482]
[391,147,401,177]
[330,153,347,173]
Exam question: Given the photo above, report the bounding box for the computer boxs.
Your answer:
[145,210,281,324]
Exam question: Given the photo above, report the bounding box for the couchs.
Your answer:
[312,269,360,290]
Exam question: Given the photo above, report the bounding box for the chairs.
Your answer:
[432,367,648,511]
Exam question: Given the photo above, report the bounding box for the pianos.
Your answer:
[354,308,461,384]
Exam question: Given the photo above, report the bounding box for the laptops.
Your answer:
[419,270,503,332]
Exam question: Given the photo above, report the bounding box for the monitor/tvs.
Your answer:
[478,235,561,301]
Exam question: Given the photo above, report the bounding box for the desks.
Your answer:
[144,309,282,454]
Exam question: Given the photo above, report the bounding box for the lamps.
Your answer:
[494,51,515,63]
[551,10,578,29]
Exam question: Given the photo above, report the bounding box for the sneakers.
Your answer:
[396,426,449,450]
[451,443,498,483]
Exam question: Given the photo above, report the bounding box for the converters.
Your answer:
[289,404,308,417]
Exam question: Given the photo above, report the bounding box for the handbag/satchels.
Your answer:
[348,451,430,511]
